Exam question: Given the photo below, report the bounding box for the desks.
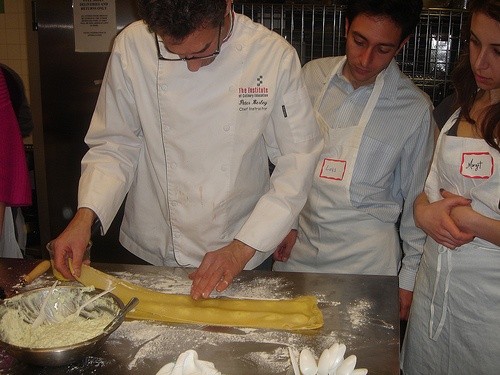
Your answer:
[0,256,400,375]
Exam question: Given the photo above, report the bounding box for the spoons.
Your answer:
[288,343,368,375]
[65,287,117,323]
[31,280,59,328]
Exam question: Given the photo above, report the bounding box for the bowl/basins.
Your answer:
[0,287,125,366]
[46,240,92,282]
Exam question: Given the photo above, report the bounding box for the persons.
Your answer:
[0,67,32,242]
[263,0,442,323]
[400,0,500,375]
[49,0,326,302]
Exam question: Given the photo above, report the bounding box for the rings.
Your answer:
[219,278,229,286]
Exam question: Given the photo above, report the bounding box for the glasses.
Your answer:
[155,22,221,61]
